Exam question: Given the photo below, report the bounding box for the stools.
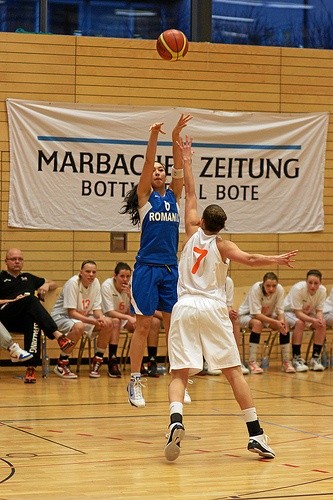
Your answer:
[40,327,333,378]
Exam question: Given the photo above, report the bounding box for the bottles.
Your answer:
[42,354,50,378]
[261,353,270,373]
[330,349,333,368]
[156,366,168,373]
[321,343,328,369]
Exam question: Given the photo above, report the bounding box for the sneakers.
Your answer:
[203,361,222,375]
[53,359,78,378]
[107,358,122,377]
[241,362,249,374]
[140,365,148,377]
[247,428,275,458]
[165,422,185,461]
[147,361,160,377]
[9,343,33,362]
[89,356,103,377]
[57,334,72,351]
[310,358,325,371]
[127,376,147,407]
[282,360,296,373]
[293,358,308,371]
[24,367,36,382]
[248,359,264,373]
[183,379,193,404]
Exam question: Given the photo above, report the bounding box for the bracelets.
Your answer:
[172,167,184,179]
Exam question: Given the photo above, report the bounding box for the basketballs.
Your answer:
[156,29,189,62]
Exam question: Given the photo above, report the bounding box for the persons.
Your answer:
[323,287,333,328]
[0,248,74,383]
[238,272,296,373]
[50,259,113,378]
[117,113,193,408]
[0,321,33,362]
[284,269,326,371]
[207,276,249,375]
[101,262,160,377]
[164,133,298,461]
